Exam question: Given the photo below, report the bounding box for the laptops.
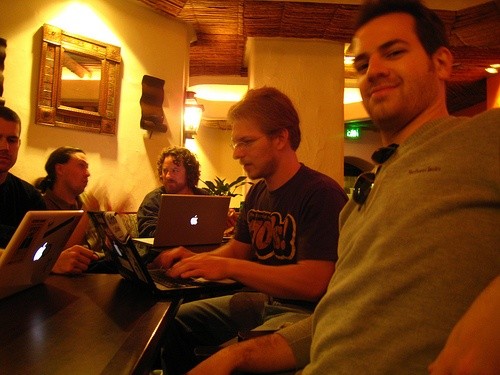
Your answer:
[86,210,245,299]
[131,194,231,247]
[0,210,85,299]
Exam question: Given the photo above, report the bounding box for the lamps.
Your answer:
[184,90,206,140]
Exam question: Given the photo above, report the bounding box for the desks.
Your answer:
[148,243,228,256]
[0,272,179,375]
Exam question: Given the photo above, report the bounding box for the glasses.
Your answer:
[232,134,270,150]
[353,143,399,212]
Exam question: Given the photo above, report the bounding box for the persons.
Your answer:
[137,147,239,238]
[150,84,349,375]
[0,106,99,276]
[35,146,122,274]
[186,1,499,375]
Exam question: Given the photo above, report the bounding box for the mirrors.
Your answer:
[34,22,122,135]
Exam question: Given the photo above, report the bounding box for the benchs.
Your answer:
[86,212,142,256]
